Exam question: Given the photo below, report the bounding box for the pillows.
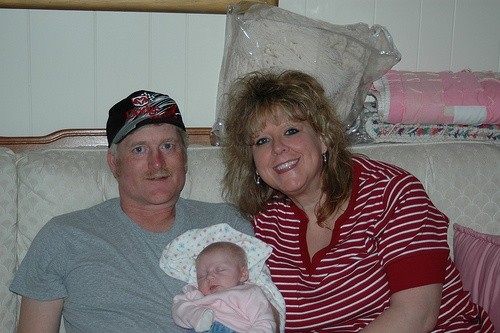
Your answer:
[453,223,500,333]
[217,18,376,145]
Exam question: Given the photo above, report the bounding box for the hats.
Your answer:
[106,90,186,148]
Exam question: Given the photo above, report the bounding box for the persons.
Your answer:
[9,89,256,333]
[223,69,497,333]
[168,241,278,333]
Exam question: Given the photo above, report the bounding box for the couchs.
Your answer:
[0,127,500,333]
